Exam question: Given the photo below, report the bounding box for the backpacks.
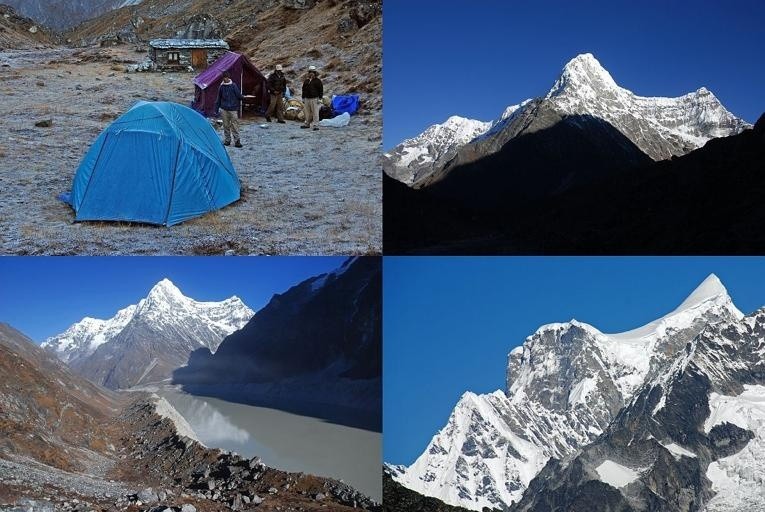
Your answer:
[319,105,331,121]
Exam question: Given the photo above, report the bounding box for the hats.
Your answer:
[275,64,282,71]
[307,66,317,72]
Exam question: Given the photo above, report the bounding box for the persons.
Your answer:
[299,66,324,132]
[214,70,246,149]
[263,64,288,125]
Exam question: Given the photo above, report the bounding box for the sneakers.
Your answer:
[278,120,285,123]
[235,140,242,148]
[301,125,310,129]
[265,113,271,122]
[313,127,319,131]
[224,141,230,146]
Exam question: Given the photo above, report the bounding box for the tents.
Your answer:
[58,97,245,231]
[191,49,270,121]
